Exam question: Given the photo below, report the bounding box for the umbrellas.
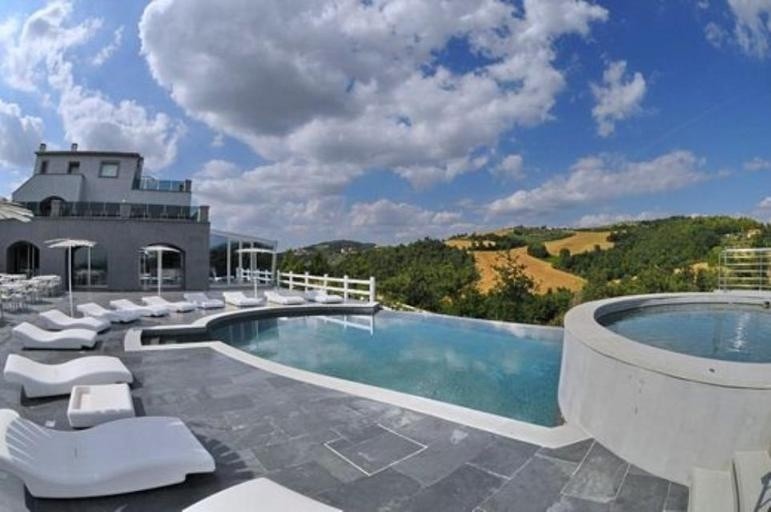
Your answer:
[1,201,34,225]
[45,238,97,321]
[234,247,277,299]
[139,244,182,299]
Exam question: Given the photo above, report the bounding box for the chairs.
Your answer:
[182,475,341,510]
[2,408,215,497]
[3,353,135,397]
[2,287,343,352]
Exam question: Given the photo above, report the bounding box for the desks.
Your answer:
[68,385,134,428]
